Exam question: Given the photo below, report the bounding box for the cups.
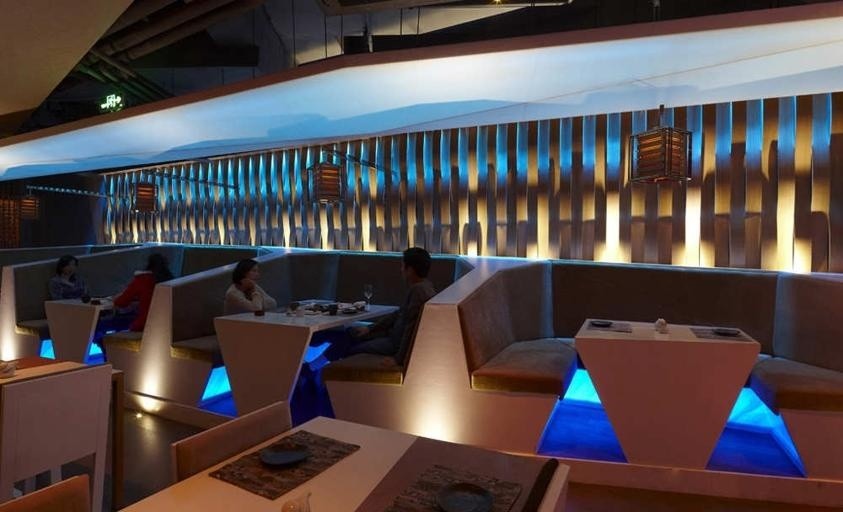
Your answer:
[253,296,264,316]
[81,296,90,303]
[327,305,338,315]
[295,305,304,317]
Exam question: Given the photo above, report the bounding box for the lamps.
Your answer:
[306,144,346,204]
[21,184,40,220]
[628,105,692,186]
[131,169,158,215]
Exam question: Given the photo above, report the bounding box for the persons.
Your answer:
[47,254,90,299]
[222,259,277,315]
[113,253,176,332]
[347,247,437,368]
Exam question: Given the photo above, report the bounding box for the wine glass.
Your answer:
[362,284,374,312]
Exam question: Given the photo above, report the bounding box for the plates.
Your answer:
[712,328,741,337]
[589,320,613,328]
[434,480,494,512]
[256,442,310,471]
